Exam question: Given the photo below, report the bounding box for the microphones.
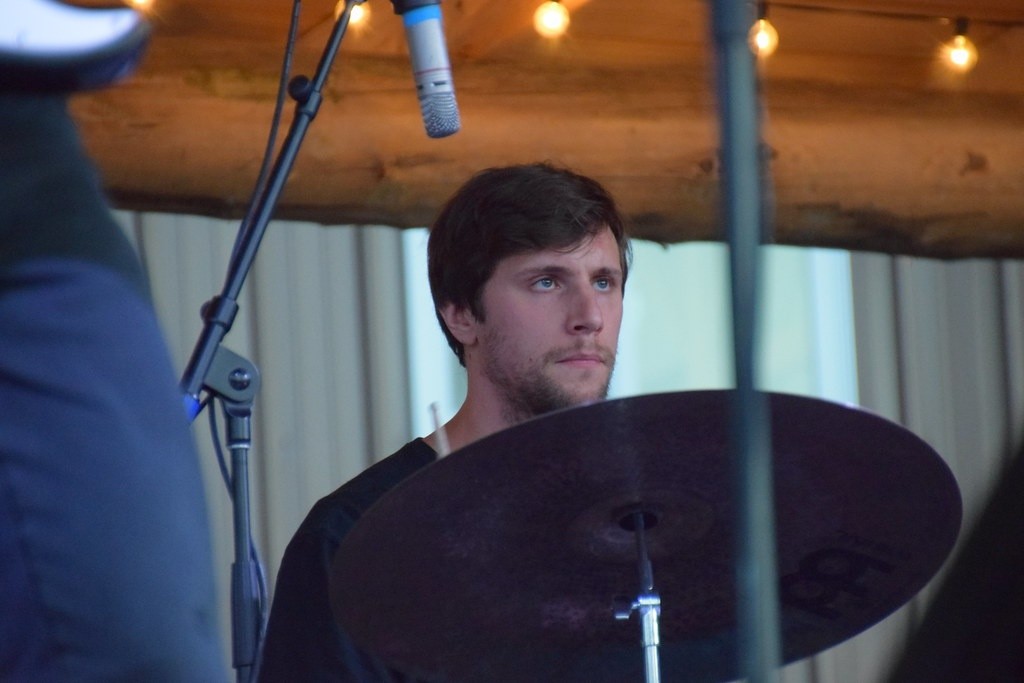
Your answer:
[392,0,464,142]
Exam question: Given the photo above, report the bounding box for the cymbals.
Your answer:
[326,387,963,683]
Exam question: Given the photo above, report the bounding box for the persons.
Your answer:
[258,159,632,683]
